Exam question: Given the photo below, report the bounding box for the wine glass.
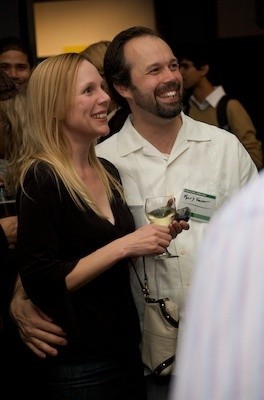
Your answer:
[145,196,180,259]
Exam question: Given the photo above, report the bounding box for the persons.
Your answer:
[10,26,261,400]
[0,36,38,400]
[178,47,262,171]
[79,41,112,116]
[16,52,189,399]
[167,172,264,400]
